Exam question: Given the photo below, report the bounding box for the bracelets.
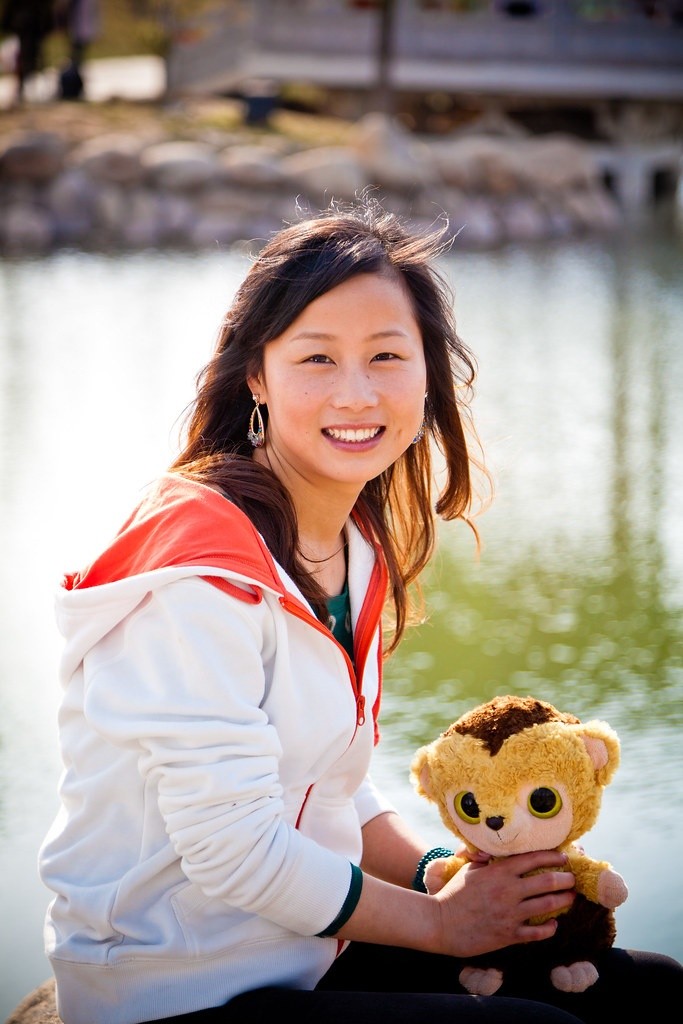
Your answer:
[412,845,459,899]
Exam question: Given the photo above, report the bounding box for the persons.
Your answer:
[41,209,682,1024]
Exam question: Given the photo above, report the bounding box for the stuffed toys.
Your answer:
[407,695,631,999]
